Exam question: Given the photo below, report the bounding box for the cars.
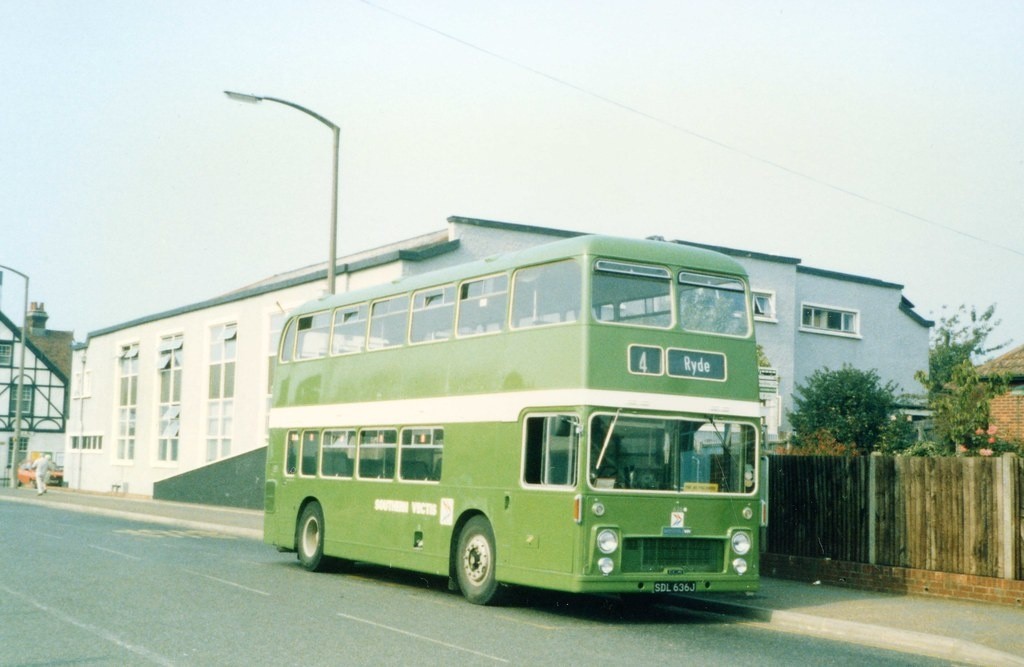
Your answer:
[17,457,64,489]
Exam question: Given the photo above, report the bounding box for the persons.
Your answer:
[32,453,49,496]
[44,455,55,493]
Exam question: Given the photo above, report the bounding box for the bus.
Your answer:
[264,234,771,611]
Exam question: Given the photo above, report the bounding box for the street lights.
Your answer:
[224,91,340,297]
